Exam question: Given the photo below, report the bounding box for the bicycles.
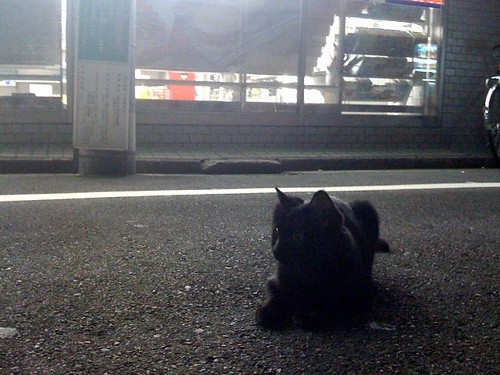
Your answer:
[484,42,500,168]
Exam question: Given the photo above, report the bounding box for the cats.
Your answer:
[255,186,391,334]
